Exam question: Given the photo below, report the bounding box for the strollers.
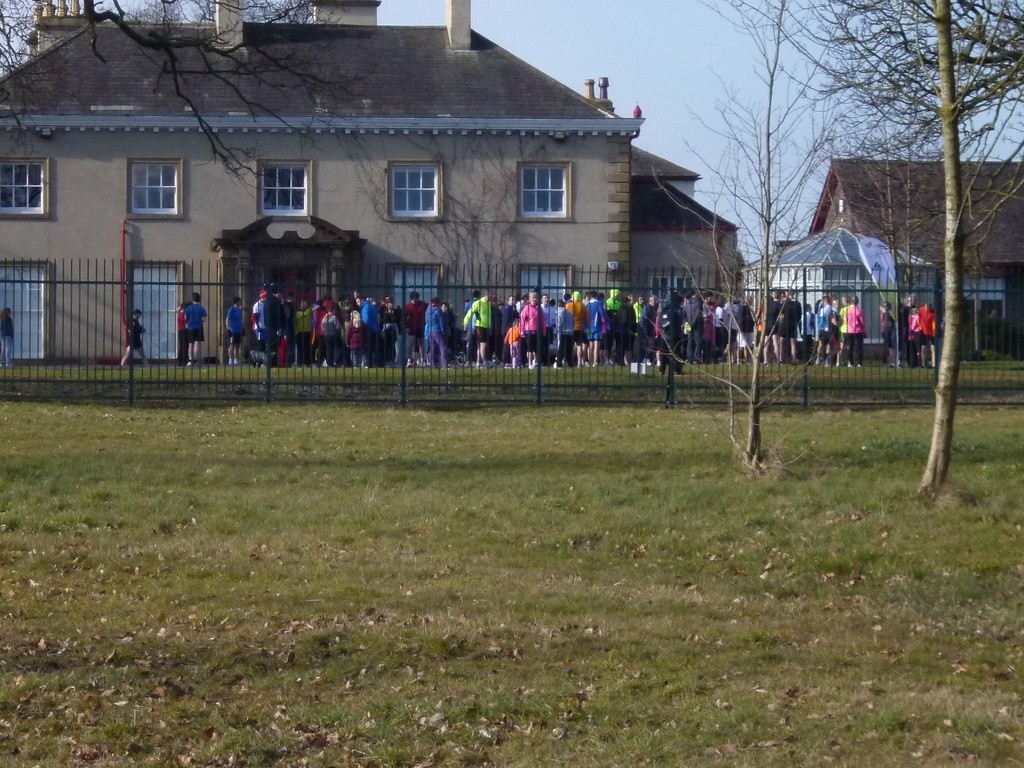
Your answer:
[446,329,466,365]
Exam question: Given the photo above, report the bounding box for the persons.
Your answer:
[119,310,149,367]
[0,309,15,368]
[175,280,938,368]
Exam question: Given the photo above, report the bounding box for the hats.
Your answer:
[259,289,267,299]
[610,289,620,299]
[572,291,581,300]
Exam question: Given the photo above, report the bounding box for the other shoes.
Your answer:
[186,361,199,366]
[0,363,13,367]
[321,359,864,367]
[229,361,239,365]
